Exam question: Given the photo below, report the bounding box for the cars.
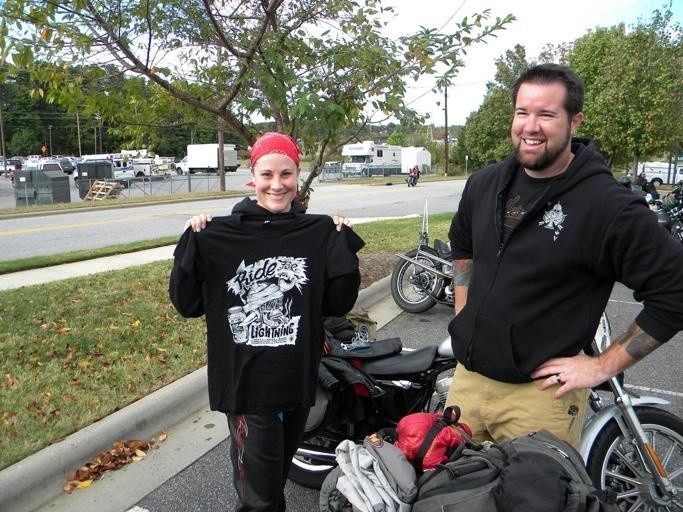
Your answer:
[0,155,81,179]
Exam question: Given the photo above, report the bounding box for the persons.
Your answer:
[437,62,683,452]
[182,132,355,511]
[637,171,646,179]
[410,165,419,175]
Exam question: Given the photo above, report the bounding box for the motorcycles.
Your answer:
[622,177,682,244]
[287,310,682,512]
[390,231,454,312]
[408,169,419,186]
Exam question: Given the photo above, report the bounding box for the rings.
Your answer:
[556,374,561,383]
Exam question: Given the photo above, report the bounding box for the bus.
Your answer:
[316,160,341,181]
[80,154,135,188]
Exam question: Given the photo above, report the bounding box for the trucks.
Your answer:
[121,144,240,180]
[634,161,683,187]
[75,158,113,200]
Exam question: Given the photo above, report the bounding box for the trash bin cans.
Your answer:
[12,164,70,207]
[77,162,112,198]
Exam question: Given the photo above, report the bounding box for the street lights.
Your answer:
[434,74,455,176]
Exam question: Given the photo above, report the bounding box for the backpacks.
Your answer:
[411,405,617,512]
